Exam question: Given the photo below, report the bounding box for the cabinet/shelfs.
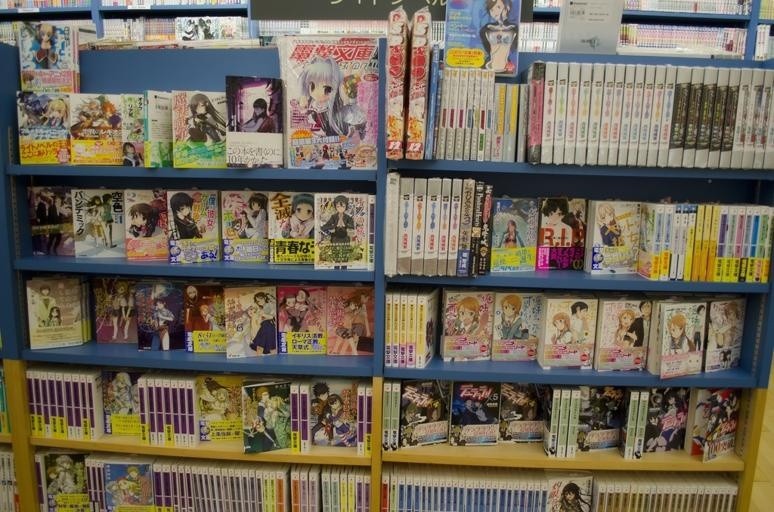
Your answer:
[0,1,773,511]
